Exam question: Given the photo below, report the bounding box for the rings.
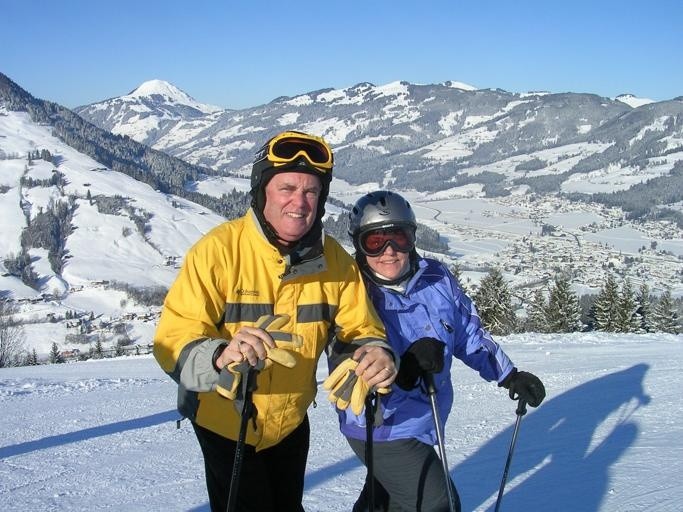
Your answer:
[384,367,392,377]
[236,341,244,352]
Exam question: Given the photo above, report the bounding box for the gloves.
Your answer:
[322,349,390,421]
[498,362,548,411]
[215,313,304,402]
[394,333,450,395]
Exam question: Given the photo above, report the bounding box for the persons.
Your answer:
[151,128,402,512]
[325,190,547,512]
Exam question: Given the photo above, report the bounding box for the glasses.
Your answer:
[353,222,416,257]
[266,132,335,172]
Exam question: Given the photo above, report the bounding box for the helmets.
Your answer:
[247,131,335,189]
[346,188,419,239]
